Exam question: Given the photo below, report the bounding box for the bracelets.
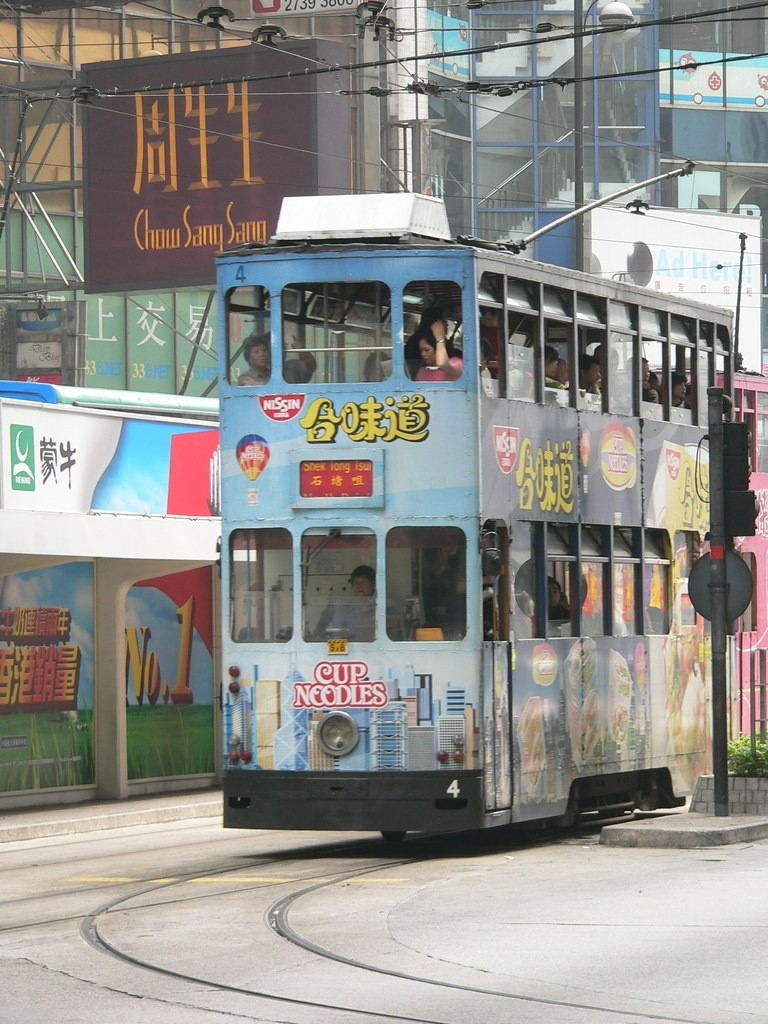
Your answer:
[435,339,446,343]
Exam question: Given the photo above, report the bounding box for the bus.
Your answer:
[651,367,768,738]
[216,193,729,841]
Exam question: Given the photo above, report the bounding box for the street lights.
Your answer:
[572,0,639,270]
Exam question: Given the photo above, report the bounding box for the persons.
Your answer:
[672,367,693,409]
[642,357,661,404]
[412,546,494,640]
[579,345,603,397]
[450,304,463,352]
[548,577,570,620]
[414,320,463,381]
[479,305,499,379]
[404,307,463,358]
[545,344,572,390]
[326,561,397,642]
[237,331,317,386]
